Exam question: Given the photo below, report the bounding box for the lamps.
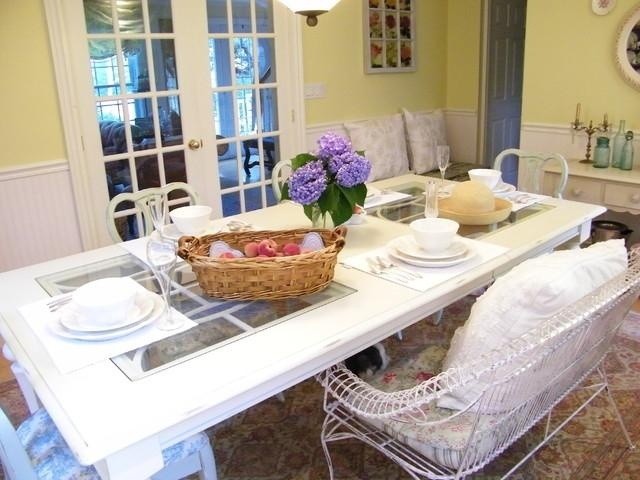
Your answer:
[274,0,342,29]
[571,98,613,166]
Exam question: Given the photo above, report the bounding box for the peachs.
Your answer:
[219,239,312,257]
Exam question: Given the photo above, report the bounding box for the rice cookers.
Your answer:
[590,220,632,244]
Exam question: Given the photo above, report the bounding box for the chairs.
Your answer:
[314,234,640,480]
[491,146,570,200]
[104,180,205,245]
[0,393,219,480]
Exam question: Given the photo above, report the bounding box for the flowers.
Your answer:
[278,131,373,228]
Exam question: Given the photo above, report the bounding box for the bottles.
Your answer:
[612,119,626,168]
[594,137,610,168]
[619,133,634,170]
[424,178,438,218]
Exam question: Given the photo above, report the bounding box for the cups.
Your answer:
[145,190,168,232]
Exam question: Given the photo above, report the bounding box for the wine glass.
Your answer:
[436,145,451,197]
[145,236,183,331]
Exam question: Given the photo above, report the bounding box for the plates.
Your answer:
[364,184,380,201]
[492,181,516,196]
[161,221,224,242]
[51,290,163,341]
[395,237,469,259]
[60,296,154,333]
[387,235,478,267]
[151,226,176,249]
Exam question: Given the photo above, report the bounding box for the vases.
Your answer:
[310,200,335,230]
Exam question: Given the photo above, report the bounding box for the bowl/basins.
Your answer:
[74,278,139,324]
[469,169,503,189]
[410,218,459,252]
[170,205,212,234]
[450,182,494,214]
[439,199,512,227]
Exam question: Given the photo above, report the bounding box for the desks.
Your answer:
[541,159,639,217]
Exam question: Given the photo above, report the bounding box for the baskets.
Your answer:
[178,224,347,302]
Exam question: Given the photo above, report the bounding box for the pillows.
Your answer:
[433,235,631,413]
[341,112,411,184]
[399,105,446,176]
